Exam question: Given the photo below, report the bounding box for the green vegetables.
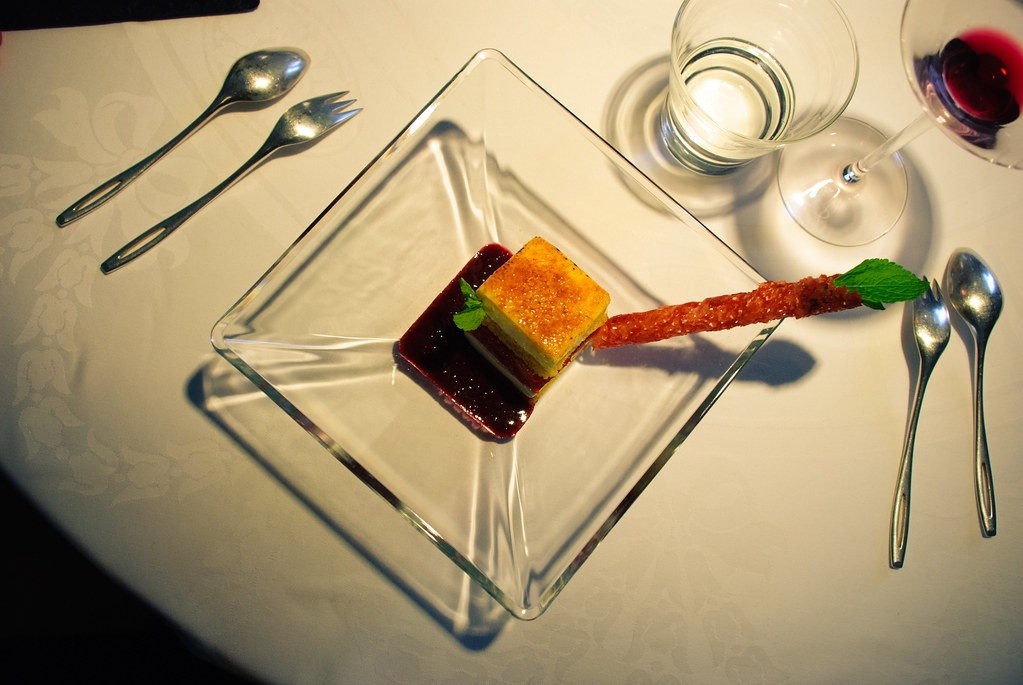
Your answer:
[452,277,487,330]
[833,258,930,311]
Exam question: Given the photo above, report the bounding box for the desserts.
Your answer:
[475,237,611,397]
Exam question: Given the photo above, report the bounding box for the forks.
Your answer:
[889,275,951,567]
[100,91,364,277]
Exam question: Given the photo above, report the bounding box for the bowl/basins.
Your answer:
[210,48,799,622]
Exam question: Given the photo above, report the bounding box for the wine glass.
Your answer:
[779,0,1023,250]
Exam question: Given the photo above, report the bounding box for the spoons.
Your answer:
[56,47,310,226]
[945,248,1003,539]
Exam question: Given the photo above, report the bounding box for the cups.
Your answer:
[655,0,860,176]
